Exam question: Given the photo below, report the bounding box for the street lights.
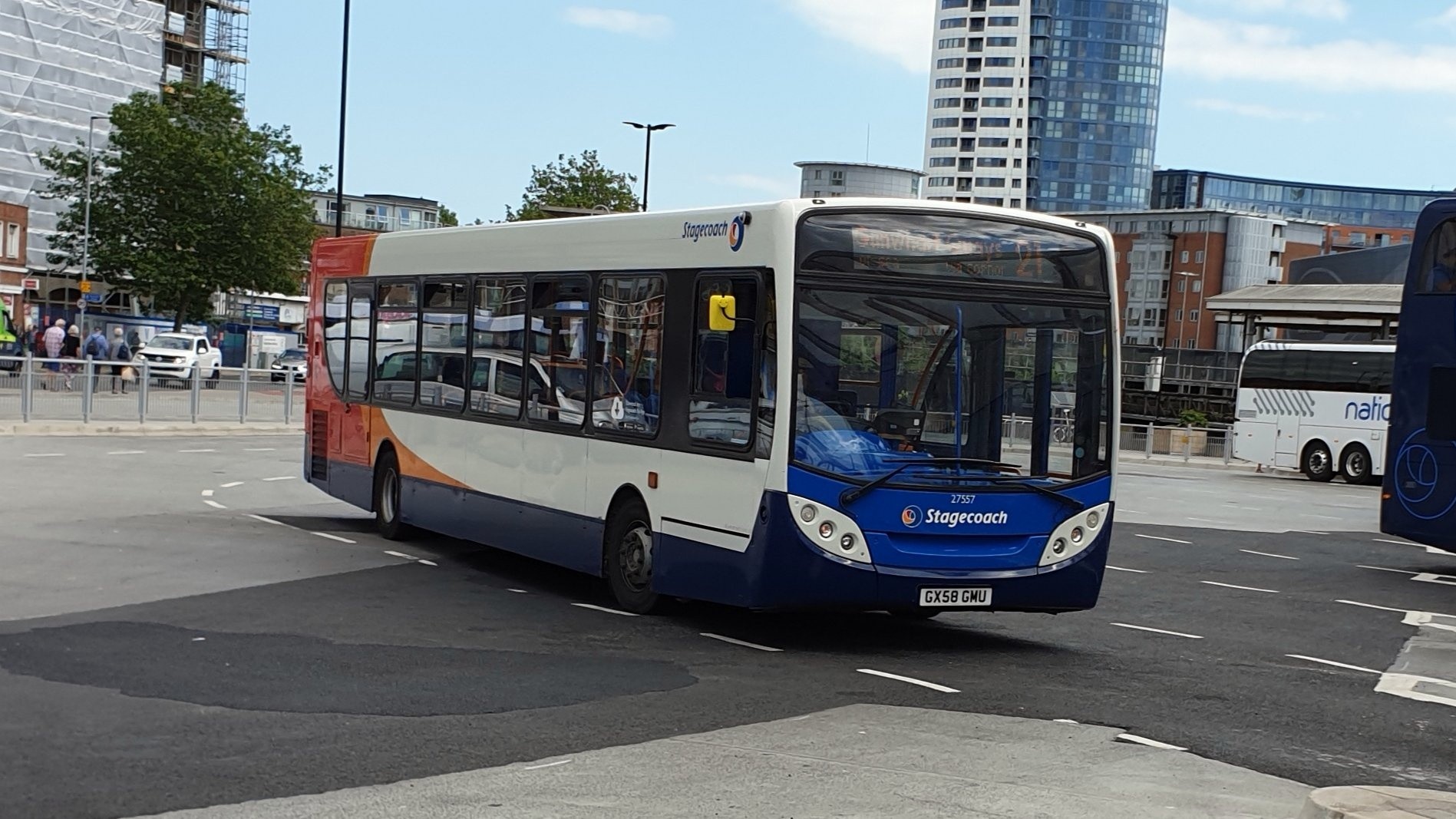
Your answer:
[1151,231,1180,421]
[1174,271,1202,377]
[77,114,116,358]
[622,120,675,211]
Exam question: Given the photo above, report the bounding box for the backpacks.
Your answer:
[88,337,100,357]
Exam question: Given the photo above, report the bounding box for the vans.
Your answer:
[365,341,651,437]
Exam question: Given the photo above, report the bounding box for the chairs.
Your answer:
[397,354,655,429]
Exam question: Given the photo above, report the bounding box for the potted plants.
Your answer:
[1153,408,1207,455]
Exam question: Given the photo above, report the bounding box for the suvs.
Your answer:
[130,332,222,388]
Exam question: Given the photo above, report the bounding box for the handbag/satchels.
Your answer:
[116,345,129,359]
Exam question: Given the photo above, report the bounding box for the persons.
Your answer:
[570,314,608,393]
[1426,246,1456,292]
[10,310,141,394]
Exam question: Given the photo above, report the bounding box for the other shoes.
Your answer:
[121,390,128,394]
[112,390,118,394]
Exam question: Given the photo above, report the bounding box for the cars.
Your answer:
[685,383,869,476]
[0,296,27,378]
[269,348,309,382]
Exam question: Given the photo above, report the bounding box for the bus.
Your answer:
[301,197,1120,624]
[1380,195,1456,557]
[1230,335,1400,483]
